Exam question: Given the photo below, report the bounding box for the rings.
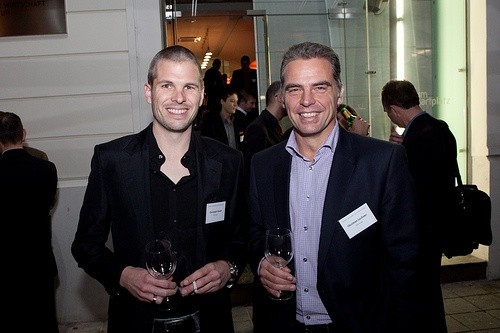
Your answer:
[153,295,157,303]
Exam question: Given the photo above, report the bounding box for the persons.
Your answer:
[336,104,370,136]
[194,55,257,151]
[70,45,246,333]
[0,111,60,333]
[248,41,417,333]
[227,81,287,310]
[382,80,457,333]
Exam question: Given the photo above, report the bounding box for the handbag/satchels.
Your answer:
[440,185,493,260]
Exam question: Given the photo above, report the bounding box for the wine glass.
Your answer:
[145,239,178,312]
[265,227,294,301]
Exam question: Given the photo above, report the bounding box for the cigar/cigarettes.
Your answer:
[193,280,199,294]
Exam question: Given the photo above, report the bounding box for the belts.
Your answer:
[150,297,233,333]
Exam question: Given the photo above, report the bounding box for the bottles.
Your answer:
[341,107,369,135]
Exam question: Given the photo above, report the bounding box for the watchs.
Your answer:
[228,262,238,285]
